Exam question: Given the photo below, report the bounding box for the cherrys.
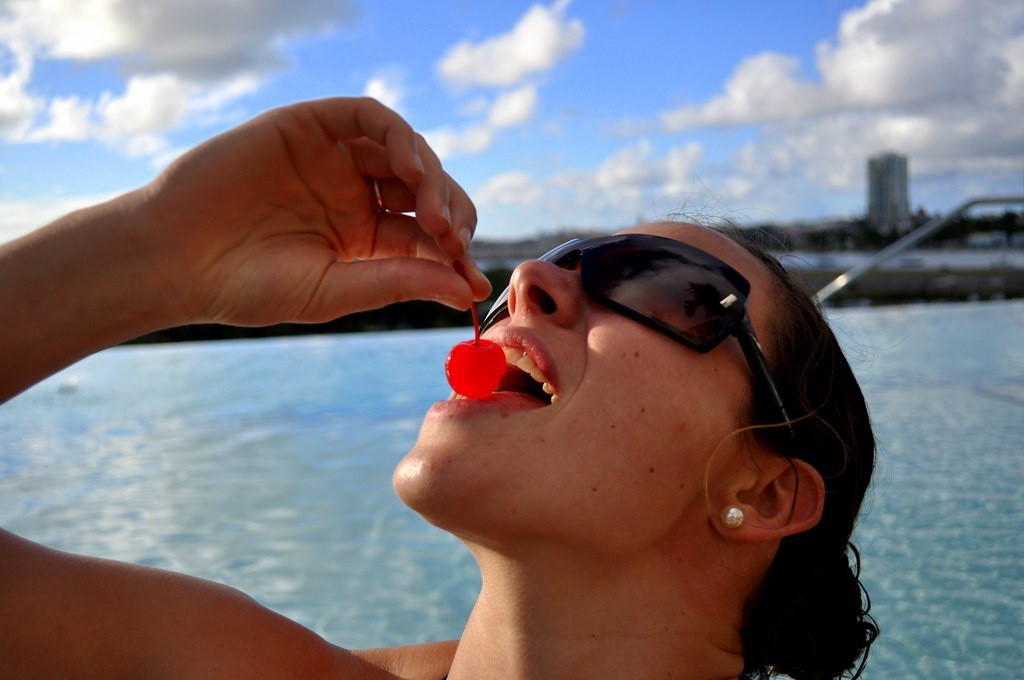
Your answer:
[445,299,507,400]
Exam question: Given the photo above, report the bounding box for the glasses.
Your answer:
[479,234,798,447]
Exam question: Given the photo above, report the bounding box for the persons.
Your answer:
[0,95,879,680]
